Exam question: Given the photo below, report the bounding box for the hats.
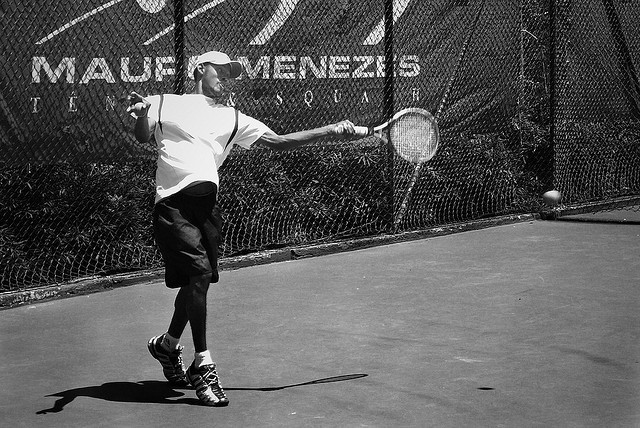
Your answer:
[193,50,243,82]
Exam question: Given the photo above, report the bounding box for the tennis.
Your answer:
[542,190,561,204]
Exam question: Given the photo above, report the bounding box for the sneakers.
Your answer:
[146,331,189,386]
[185,358,230,406]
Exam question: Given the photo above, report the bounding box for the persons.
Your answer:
[126,50,356,406]
